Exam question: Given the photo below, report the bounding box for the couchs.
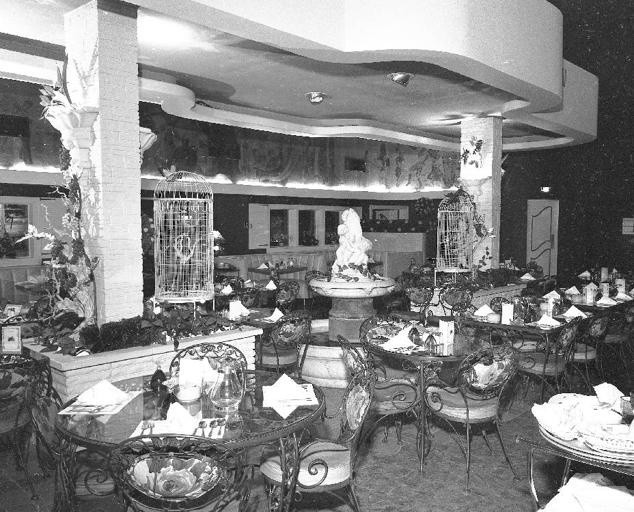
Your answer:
[220,252,325,306]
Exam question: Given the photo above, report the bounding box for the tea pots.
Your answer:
[172,382,201,403]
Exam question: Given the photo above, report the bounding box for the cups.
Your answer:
[621,396,634,425]
[560,406,567,424]
[603,284,609,300]
[502,304,513,326]
[602,268,608,283]
[584,288,595,305]
[541,303,553,319]
[616,281,625,296]
[575,408,583,421]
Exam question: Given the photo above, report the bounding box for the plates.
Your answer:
[584,440,634,457]
[586,437,634,453]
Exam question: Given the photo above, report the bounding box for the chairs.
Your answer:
[568,313,612,393]
[258,312,312,377]
[423,341,520,489]
[489,296,510,312]
[335,334,431,455]
[359,312,422,355]
[258,356,377,510]
[2,356,53,502]
[438,283,479,317]
[160,342,251,397]
[452,302,504,348]
[107,435,258,511]
[519,315,584,402]
[406,284,439,316]
[273,280,299,312]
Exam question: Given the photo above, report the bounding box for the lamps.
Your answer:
[391,71,416,87]
[307,93,325,105]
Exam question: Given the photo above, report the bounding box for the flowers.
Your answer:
[39,52,81,109]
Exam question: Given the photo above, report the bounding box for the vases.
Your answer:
[45,107,96,188]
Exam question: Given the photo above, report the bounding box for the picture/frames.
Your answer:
[2,204,30,258]
[1,326,21,356]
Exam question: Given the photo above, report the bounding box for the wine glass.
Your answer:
[407,320,420,337]
[453,321,466,357]
[210,367,243,423]
[423,331,437,355]
